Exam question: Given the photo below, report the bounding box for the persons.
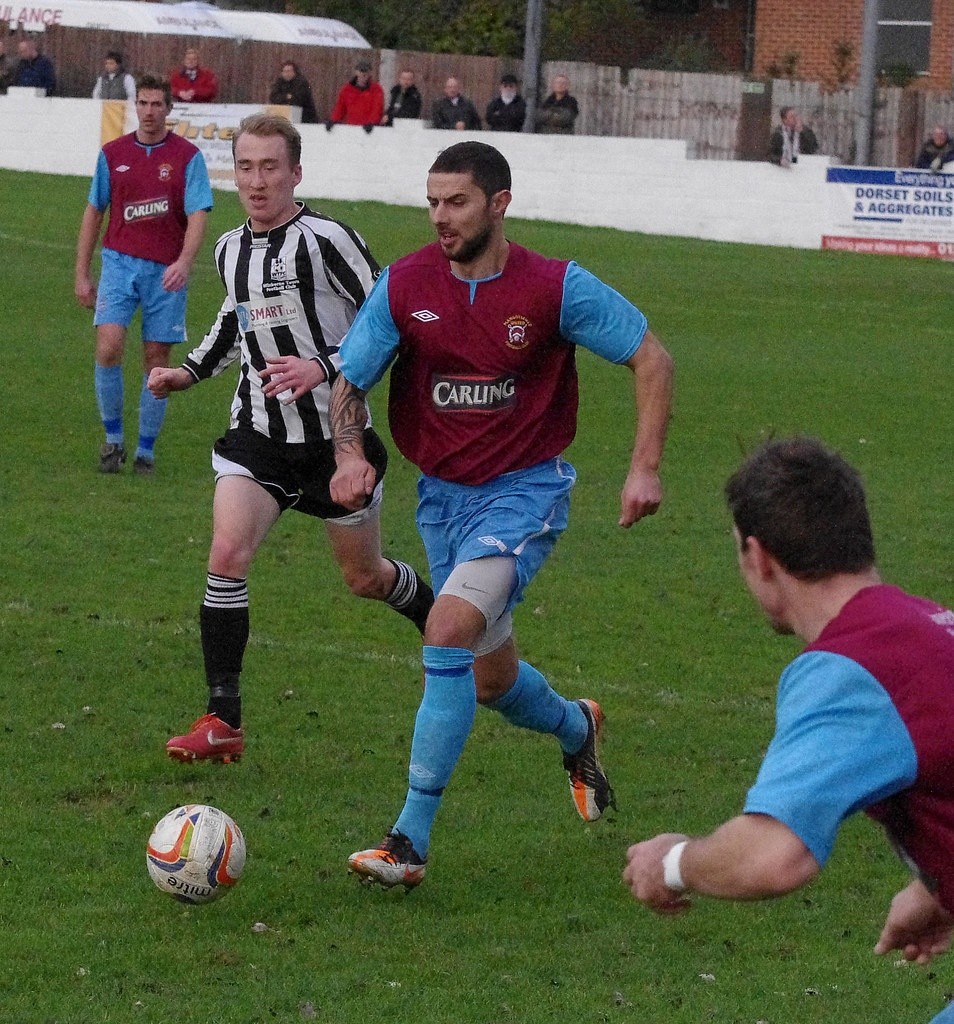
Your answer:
[146,114,433,762]
[430,78,482,130]
[532,73,579,134]
[269,60,318,124]
[376,68,422,126]
[767,107,818,168]
[619,437,954,1024]
[325,62,384,134]
[327,141,674,898]
[91,51,137,103]
[168,48,220,103]
[915,126,954,172]
[484,74,526,132]
[74,71,213,477]
[0,37,57,97]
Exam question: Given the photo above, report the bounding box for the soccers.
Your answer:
[146,804,246,905]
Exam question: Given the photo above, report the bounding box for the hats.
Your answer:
[501,73,517,85]
[355,62,373,72]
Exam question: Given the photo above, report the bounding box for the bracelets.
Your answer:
[662,840,690,894]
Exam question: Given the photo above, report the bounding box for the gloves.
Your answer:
[363,123,374,134]
[326,121,334,131]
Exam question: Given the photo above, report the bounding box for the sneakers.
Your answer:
[563,699,610,823]
[346,833,427,894]
[97,444,127,474]
[164,714,248,764]
[135,457,154,474]
